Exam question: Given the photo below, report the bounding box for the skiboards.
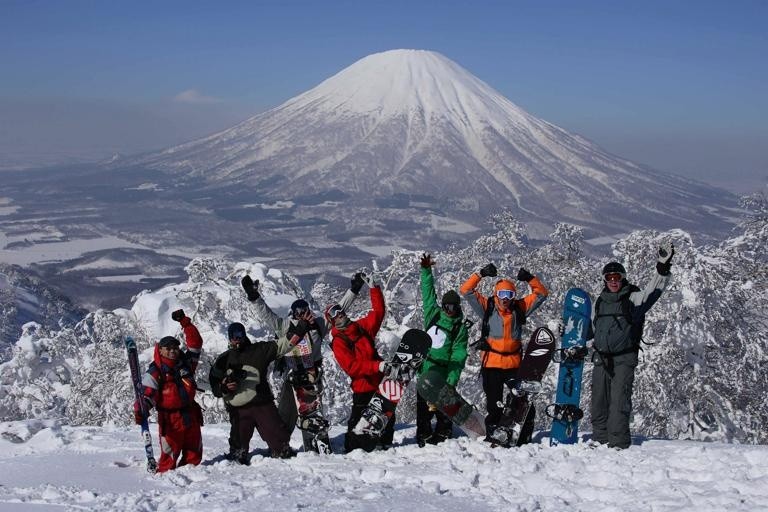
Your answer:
[123,338,157,475]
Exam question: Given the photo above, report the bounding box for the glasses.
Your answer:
[496,290,516,301]
[295,305,310,317]
[442,303,461,313]
[602,271,626,282]
[230,330,244,340]
[328,304,344,319]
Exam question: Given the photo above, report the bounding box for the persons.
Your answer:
[558,238,674,449]
[459,264,548,444]
[241,274,367,455]
[209,310,316,465]
[415,254,468,445]
[134,308,203,473]
[322,268,396,451]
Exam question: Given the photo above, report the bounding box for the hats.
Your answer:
[602,262,626,274]
[442,290,460,304]
[325,304,337,322]
[291,300,308,311]
[495,279,517,292]
[228,323,245,331]
[159,336,180,347]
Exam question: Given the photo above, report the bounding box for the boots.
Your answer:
[417,421,453,444]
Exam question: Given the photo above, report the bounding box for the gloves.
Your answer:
[518,268,533,283]
[420,253,436,268]
[351,273,366,294]
[480,263,497,277]
[172,309,185,321]
[242,275,260,301]
[380,361,401,380]
[361,274,376,288]
[657,241,674,271]
[134,401,150,424]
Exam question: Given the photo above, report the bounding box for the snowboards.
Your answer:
[416,370,487,439]
[550,287,592,444]
[284,322,333,455]
[345,329,432,452]
[489,327,556,448]
